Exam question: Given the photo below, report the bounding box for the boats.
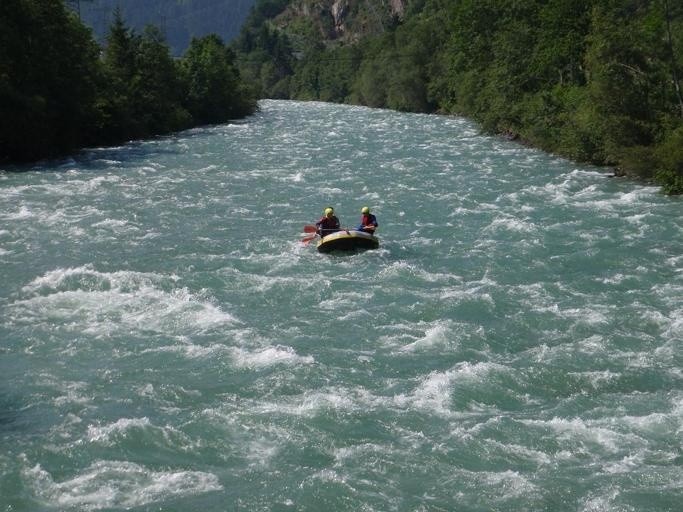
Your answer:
[313,230,383,254]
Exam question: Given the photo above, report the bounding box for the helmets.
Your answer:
[324,208,333,215]
[361,207,370,214]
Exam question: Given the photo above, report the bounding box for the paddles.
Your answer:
[304,224,344,233]
[302,235,315,244]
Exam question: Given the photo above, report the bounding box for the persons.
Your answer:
[355,206,377,235]
[315,207,339,238]
